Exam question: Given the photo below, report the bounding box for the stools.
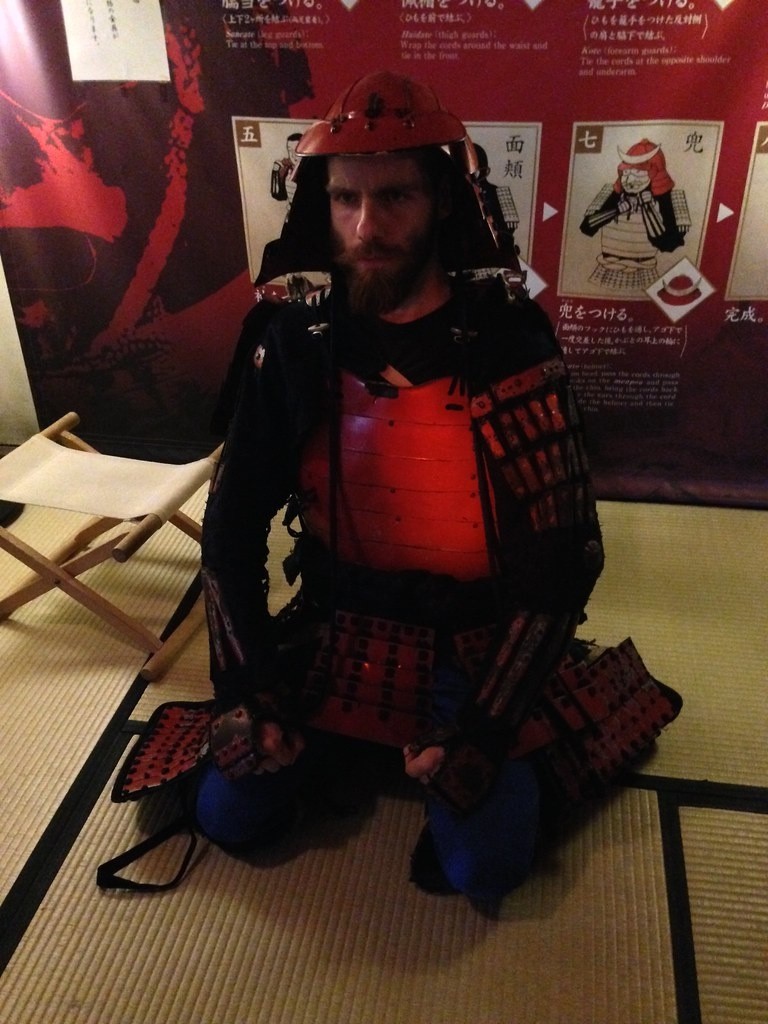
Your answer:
[0,410,228,684]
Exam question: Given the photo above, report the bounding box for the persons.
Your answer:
[93,68,685,919]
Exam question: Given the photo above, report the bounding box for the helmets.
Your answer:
[289,71,479,182]
